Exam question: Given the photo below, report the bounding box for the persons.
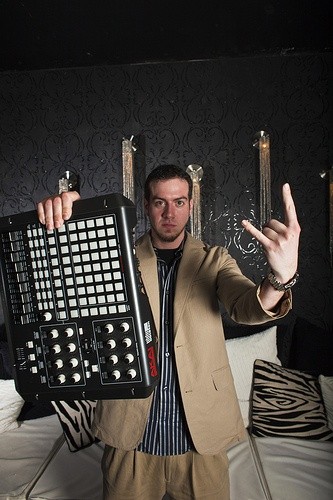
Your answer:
[38,164,301,500]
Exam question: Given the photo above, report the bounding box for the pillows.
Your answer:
[226,326,281,429]
[250,359,333,442]
[50,400,101,453]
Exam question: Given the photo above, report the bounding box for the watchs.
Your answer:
[266,268,299,292]
